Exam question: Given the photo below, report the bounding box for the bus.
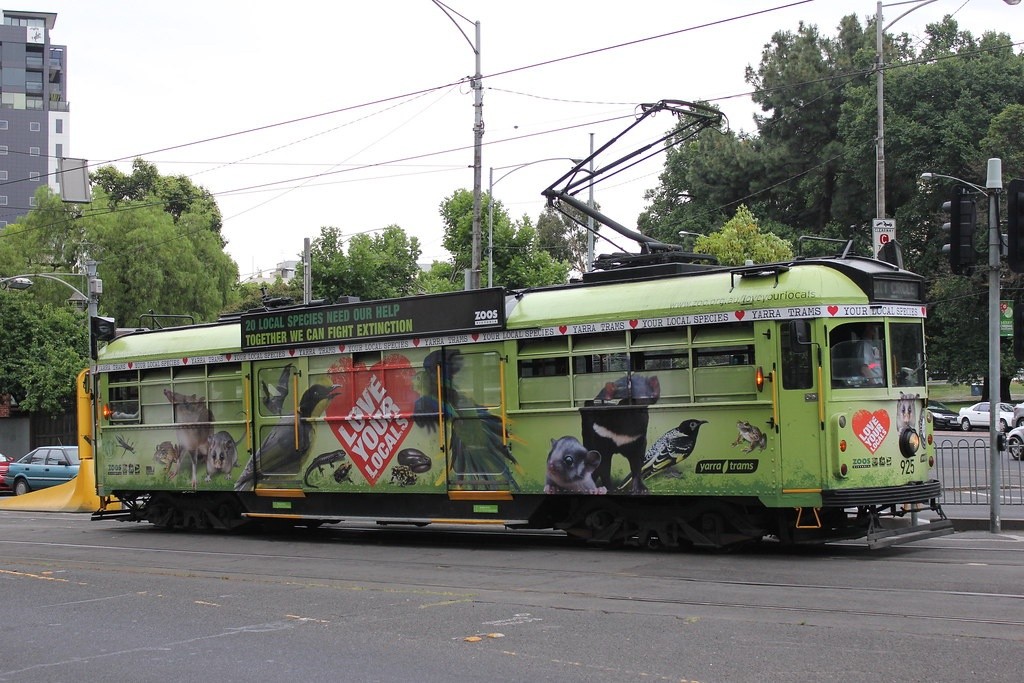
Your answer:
[83,100,957,553]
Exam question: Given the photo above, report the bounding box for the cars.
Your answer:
[927,399,962,430]
[5,445,84,497]
[957,401,1014,432]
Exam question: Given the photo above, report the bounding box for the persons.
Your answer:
[850,325,882,385]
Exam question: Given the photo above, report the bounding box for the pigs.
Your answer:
[578,373,662,494]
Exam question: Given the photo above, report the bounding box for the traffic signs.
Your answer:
[872,219,896,260]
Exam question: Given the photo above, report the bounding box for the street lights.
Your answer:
[3,262,103,450]
[920,159,1004,529]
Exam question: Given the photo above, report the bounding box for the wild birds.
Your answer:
[616,419,709,491]
[407,349,528,492]
[260,362,294,416]
[233,383,342,491]
